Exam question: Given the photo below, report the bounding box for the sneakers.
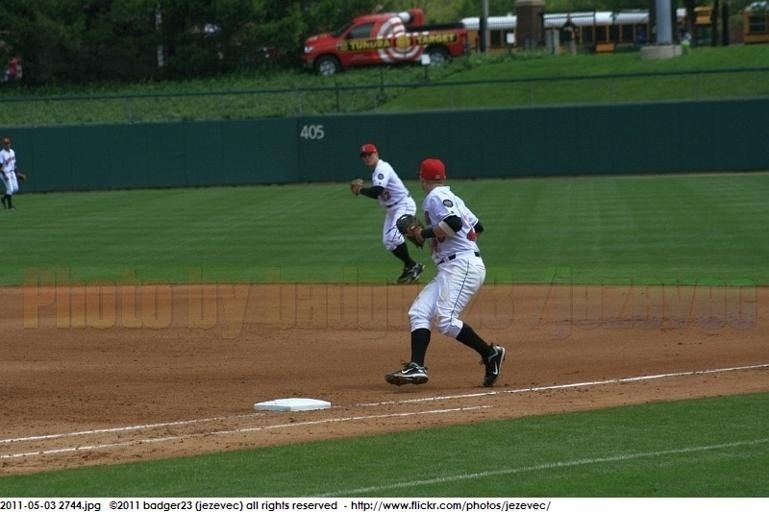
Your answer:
[2,138,12,145]
[397,263,427,284]
[482,345,505,387]
[1,197,6,208]
[385,361,428,386]
[8,206,16,209]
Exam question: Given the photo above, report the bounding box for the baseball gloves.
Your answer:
[16,171,26,181]
[349,178,363,196]
[396,214,425,249]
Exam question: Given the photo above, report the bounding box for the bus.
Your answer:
[460,8,691,50]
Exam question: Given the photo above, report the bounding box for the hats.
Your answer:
[419,158,447,180]
[360,144,377,157]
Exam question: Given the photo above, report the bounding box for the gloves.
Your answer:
[17,172,26,181]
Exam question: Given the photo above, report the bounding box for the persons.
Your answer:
[385,158,505,392]
[0,137,25,210]
[348,142,426,285]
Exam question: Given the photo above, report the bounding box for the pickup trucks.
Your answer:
[301,7,467,76]
